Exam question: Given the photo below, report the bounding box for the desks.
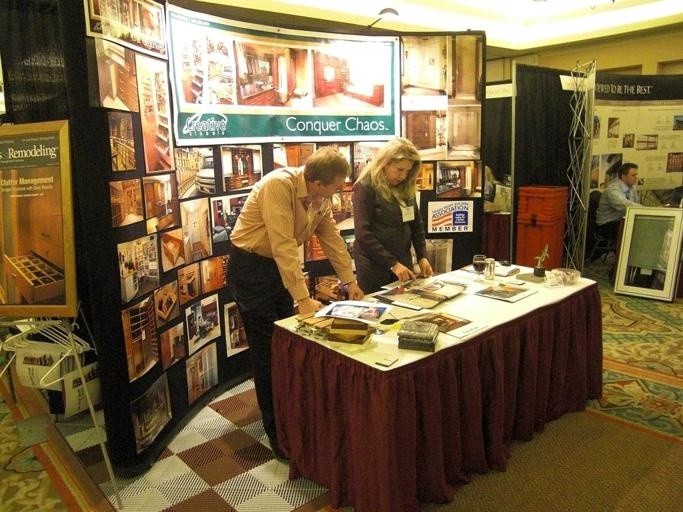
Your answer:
[482,210,510,263]
[274,259,599,512]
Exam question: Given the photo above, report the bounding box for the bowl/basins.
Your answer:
[552,268,581,286]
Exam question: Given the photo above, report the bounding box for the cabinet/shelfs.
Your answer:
[2,166,65,305]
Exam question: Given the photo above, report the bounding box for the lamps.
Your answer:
[357,6,399,33]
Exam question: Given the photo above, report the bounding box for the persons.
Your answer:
[350,137,435,294]
[233,153,251,175]
[594,164,643,237]
[224,146,366,464]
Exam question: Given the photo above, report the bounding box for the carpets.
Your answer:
[51,374,356,512]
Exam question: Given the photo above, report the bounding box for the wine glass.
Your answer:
[472,254,486,283]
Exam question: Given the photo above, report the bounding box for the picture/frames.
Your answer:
[613,204,683,302]
[0,119,81,318]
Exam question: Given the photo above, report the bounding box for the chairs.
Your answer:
[587,190,617,262]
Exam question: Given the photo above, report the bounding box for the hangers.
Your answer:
[2,314,99,388]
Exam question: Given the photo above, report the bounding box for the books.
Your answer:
[326,318,375,345]
[397,320,440,352]
[373,274,468,309]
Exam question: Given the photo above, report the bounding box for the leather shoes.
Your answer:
[608,269,615,287]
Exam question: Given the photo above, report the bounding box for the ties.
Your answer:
[625,189,631,200]
